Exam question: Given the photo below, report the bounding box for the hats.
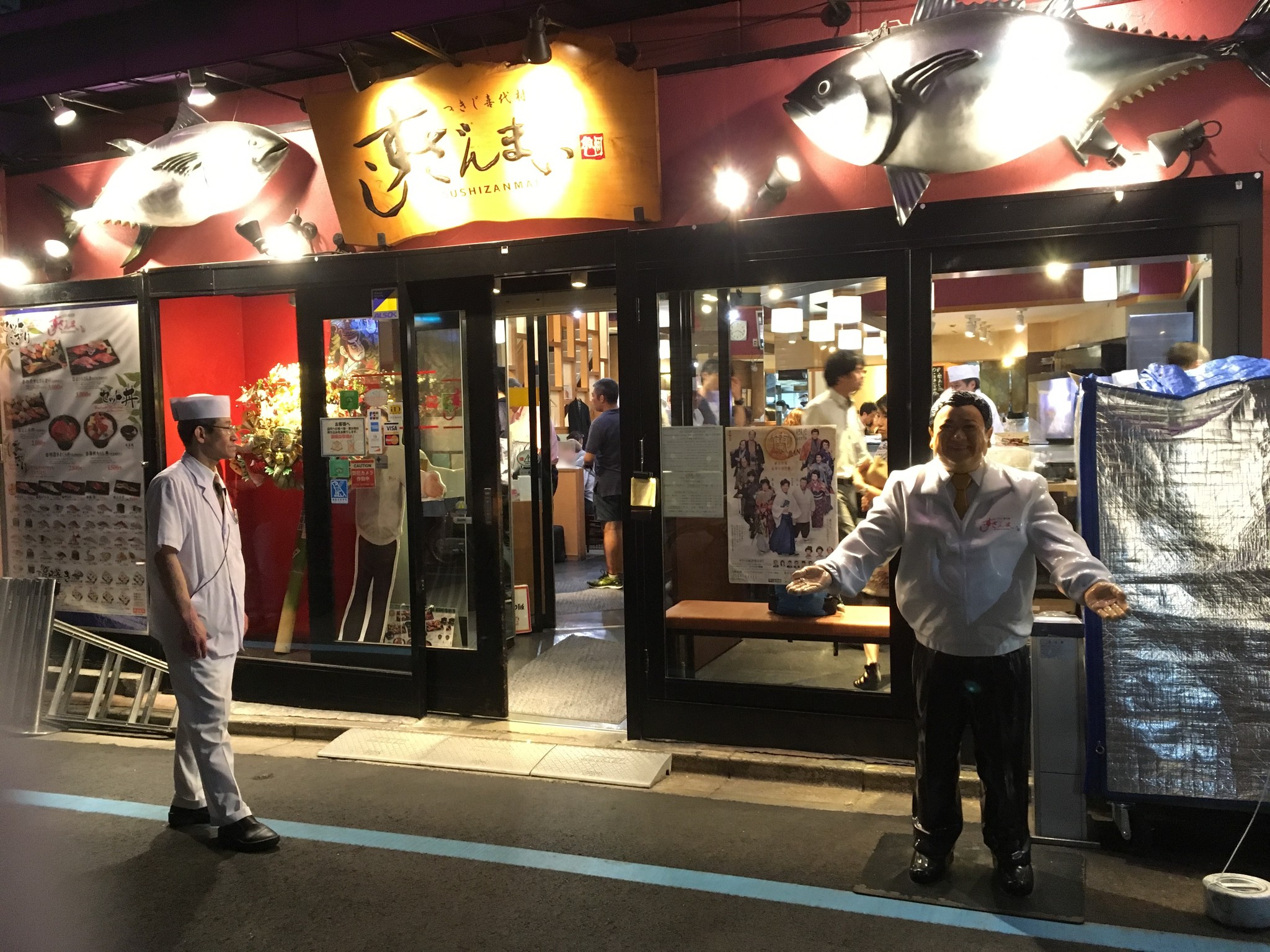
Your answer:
[169,394,231,421]
[947,361,980,383]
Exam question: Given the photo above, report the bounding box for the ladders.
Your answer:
[39,618,181,736]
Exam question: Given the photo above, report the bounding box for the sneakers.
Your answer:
[587,568,623,589]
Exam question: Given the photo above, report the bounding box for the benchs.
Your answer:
[666,597,890,680]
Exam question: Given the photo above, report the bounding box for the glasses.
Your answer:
[202,424,236,435]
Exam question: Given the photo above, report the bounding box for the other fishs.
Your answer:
[41,98,292,268]
[781,0,1270,227]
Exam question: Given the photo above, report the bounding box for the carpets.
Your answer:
[556,588,624,615]
[508,634,627,725]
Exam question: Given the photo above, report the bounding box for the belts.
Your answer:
[837,478,853,486]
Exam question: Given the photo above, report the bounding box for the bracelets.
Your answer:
[735,400,744,405]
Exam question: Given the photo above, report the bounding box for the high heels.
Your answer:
[853,662,882,691]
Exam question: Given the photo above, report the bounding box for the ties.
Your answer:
[213,473,224,516]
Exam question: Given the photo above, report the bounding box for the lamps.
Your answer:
[1146,119,1205,168]
[570,271,588,287]
[1076,121,1132,168]
[770,289,887,360]
[754,155,801,215]
[820,2,852,28]
[42,93,77,127]
[280,207,317,240]
[339,41,380,93]
[522,15,564,64]
[235,217,275,258]
[492,277,502,294]
[965,308,1028,341]
[44,237,77,257]
[187,67,217,107]
[288,293,296,306]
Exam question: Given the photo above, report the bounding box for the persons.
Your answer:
[342,409,444,642]
[730,429,834,568]
[666,358,745,426]
[785,388,1129,899]
[1167,341,1199,369]
[143,394,280,850]
[508,378,559,497]
[947,364,1003,433]
[801,349,890,690]
[583,378,623,589]
[564,431,595,515]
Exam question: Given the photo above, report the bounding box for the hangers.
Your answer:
[576,388,581,402]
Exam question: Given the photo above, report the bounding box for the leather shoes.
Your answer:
[217,815,279,851]
[167,803,209,826]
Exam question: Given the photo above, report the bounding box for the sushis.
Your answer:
[11,407,49,423]
[69,340,117,368]
[12,482,145,605]
[19,340,63,373]
